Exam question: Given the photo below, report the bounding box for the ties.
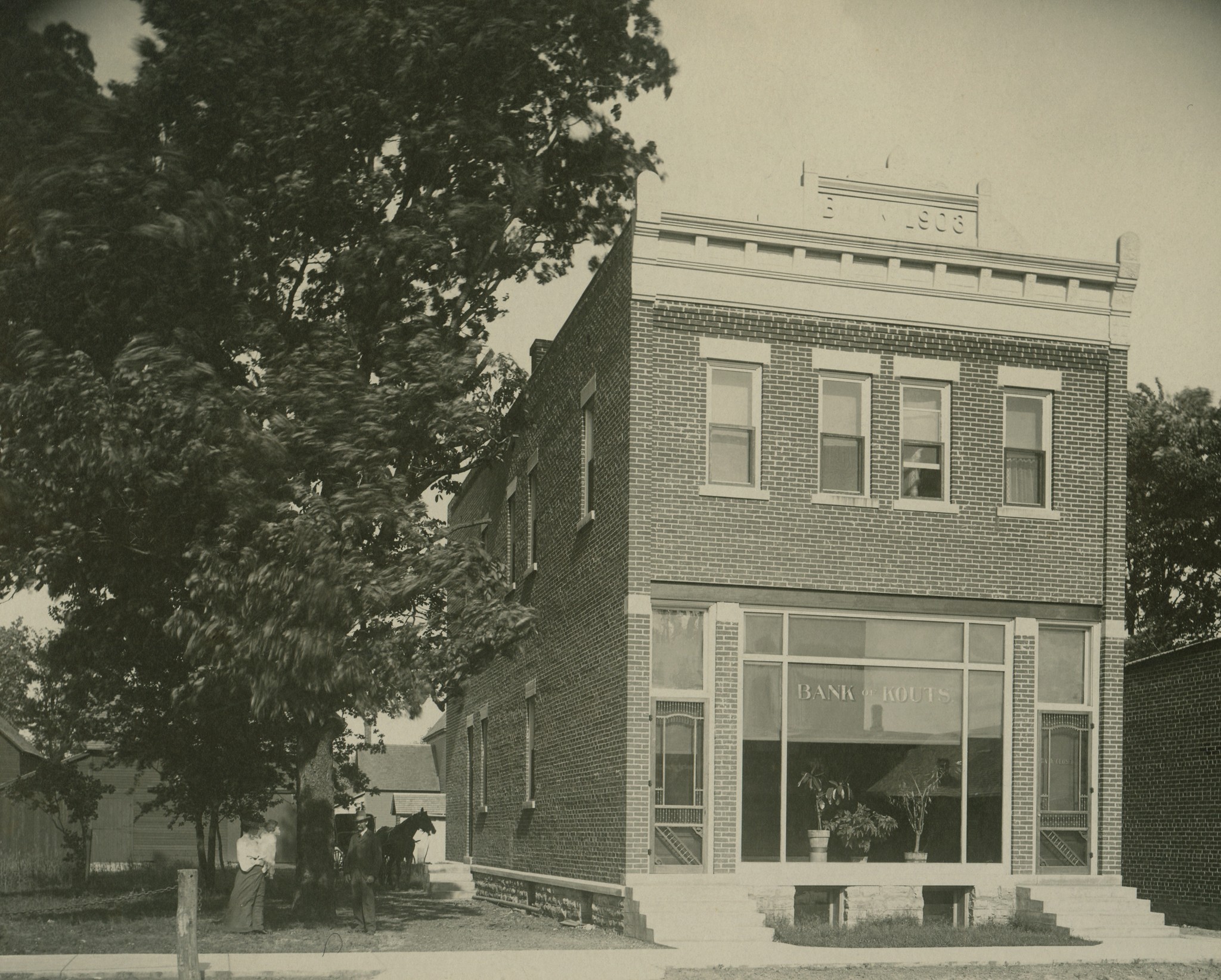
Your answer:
[358,833,361,839]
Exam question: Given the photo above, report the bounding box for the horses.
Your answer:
[374,807,437,890]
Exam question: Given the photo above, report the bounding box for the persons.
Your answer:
[252,819,281,879]
[220,812,271,935]
[345,809,383,936]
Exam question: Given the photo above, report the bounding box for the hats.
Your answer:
[351,811,371,822]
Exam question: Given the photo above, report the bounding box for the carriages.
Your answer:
[333,806,438,891]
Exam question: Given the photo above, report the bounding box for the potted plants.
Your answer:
[885,756,963,863]
[823,800,899,863]
[796,765,854,862]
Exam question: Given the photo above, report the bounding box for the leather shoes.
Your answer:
[253,929,271,935]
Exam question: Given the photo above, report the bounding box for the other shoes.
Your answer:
[348,929,367,934]
[366,931,375,936]
